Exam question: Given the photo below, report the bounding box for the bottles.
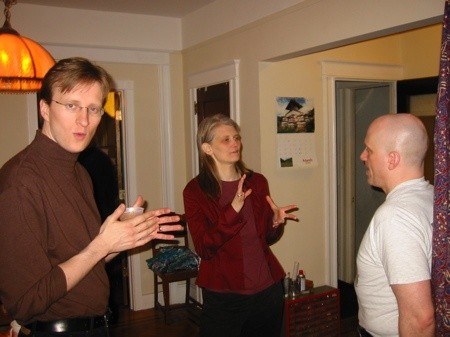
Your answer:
[296,270,306,291]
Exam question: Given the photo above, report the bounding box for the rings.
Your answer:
[159,225,161,231]
[236,194,242,196]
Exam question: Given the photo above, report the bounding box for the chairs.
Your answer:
[151,211,206,326]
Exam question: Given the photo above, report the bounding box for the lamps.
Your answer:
[0,0,57,95]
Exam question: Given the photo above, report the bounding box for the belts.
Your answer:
[23,306,113,337]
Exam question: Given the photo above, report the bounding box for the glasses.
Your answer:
[50,98,105,117]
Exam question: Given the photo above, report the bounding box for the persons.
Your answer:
[0,57,183,337]
[183,113,298,337]
[354,113,435,337]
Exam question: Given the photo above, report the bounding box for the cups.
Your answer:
[120,207,144,221]
